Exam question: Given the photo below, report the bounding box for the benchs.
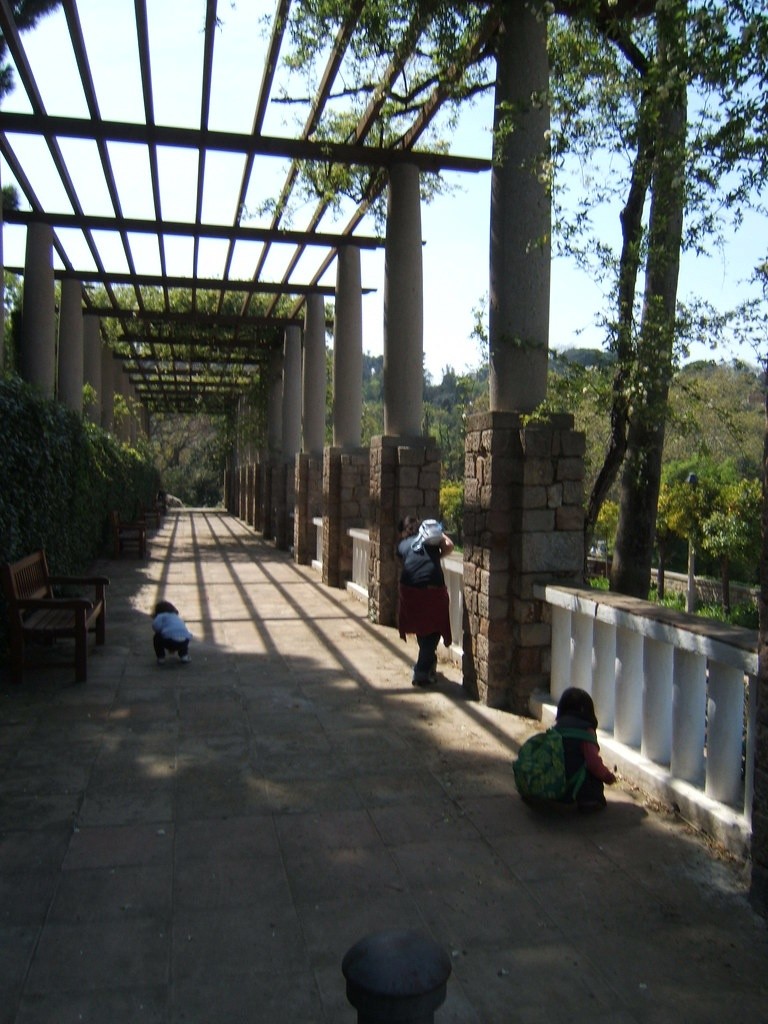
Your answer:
[0,548,111,682]
[137,495,161,529]
[111,510,147,558]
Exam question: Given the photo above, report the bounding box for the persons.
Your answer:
[521,688,617,816]
[395,514,455,688]
[150,601,193,665]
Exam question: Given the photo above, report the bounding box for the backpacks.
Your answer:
[411,518,442,553]
[513,727,598,799]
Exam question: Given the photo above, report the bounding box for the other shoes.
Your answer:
[180,654,192,662]
[158,658,166,664]
[410,678,434,685]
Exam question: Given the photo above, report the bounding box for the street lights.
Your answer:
[684,472,699,613]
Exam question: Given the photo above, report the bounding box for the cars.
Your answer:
[589,540,606,558]
